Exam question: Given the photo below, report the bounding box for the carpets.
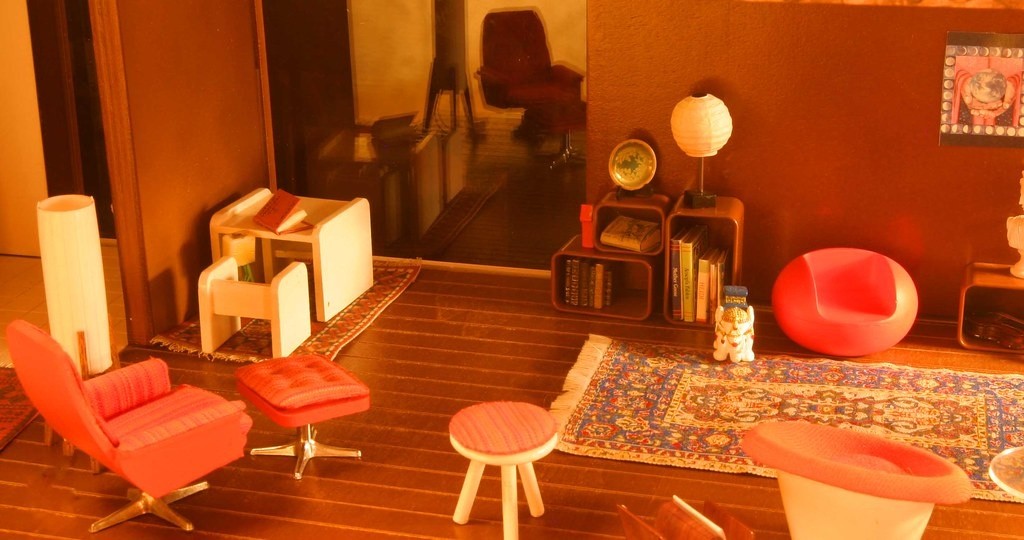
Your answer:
[0,367,42,451]
[150,260,431,361]
[544,332,1024,503]
[376,168,509,258]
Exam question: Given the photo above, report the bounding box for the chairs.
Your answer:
[743,412,976,540]
[772,248,920,357]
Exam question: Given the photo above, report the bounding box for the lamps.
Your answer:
[670,92,734,208]
[37,195,116,477]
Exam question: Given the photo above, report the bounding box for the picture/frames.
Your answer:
[939,31,1024,148]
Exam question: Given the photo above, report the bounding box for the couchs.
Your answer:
[6,318,255,533]
[479,10,588,162]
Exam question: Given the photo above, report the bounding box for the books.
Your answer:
[254,189,313,236]
[564,214,725,326]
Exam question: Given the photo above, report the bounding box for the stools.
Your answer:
[236,354,371,480]
[197,256,313,359]
[448,400,559,540]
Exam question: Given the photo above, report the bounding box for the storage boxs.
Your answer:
[957,262,1024,354]
[665,192,745,330]
[551,232,655,323]
[589,191,670,256]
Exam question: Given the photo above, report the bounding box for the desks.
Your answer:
[306,121,443,243]
[421,0,474,132]
[208,187,374,322]
